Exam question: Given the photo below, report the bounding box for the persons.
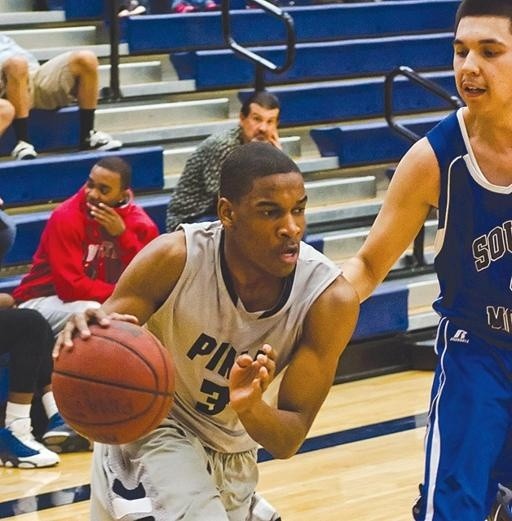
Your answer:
[1,30,125,164]
[48,141,361,521]
[0,197,91,471]
[335,1,511,521]
[164,89,284,232]
[9,155,162,339]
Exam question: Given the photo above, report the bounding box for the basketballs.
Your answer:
[51,320,177,445]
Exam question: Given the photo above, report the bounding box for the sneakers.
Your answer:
[1,412,93,468]
[85,128,122,151]
[11,139,37,160]
